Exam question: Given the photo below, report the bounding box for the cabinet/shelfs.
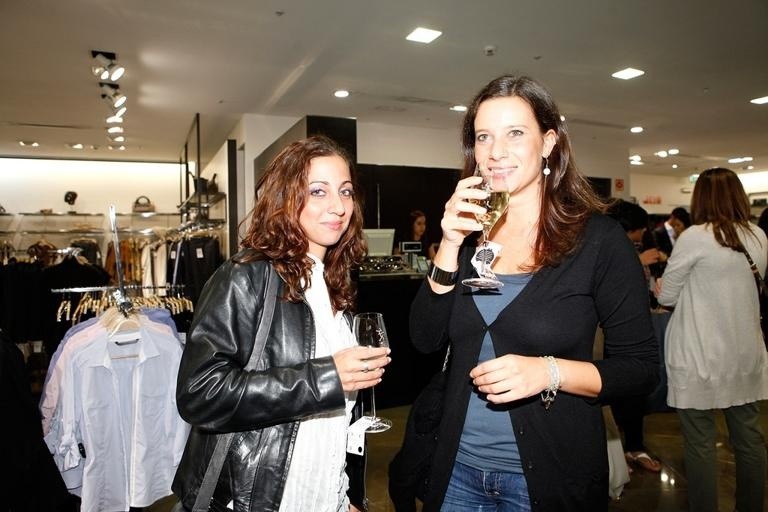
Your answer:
[176,113,226,235]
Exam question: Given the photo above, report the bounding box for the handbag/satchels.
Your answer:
[132,196,155,212]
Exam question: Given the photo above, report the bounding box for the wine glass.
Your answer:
[350,312,393,434]
[461,162,510,290]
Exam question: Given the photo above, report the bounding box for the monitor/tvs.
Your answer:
[361,228,395,257]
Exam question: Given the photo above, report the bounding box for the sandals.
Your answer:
[625,452,661,473]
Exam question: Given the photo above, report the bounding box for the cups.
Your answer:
[358,254,403,274]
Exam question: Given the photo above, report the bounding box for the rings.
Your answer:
[363,359,369,373]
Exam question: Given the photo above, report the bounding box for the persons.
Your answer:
[651,207,690,276]
[407,73,664,512]
[672,213,690,241]
[602,201,663,476]
[652,165,768,510]
[170,131,393,511]
[391,210,436,260]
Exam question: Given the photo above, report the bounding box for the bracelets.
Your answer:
[540,354,561,410]
[427,263,459,286]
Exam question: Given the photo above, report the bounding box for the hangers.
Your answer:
[54,283,193,350]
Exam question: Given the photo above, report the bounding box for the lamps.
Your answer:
[89,49,126,118]
